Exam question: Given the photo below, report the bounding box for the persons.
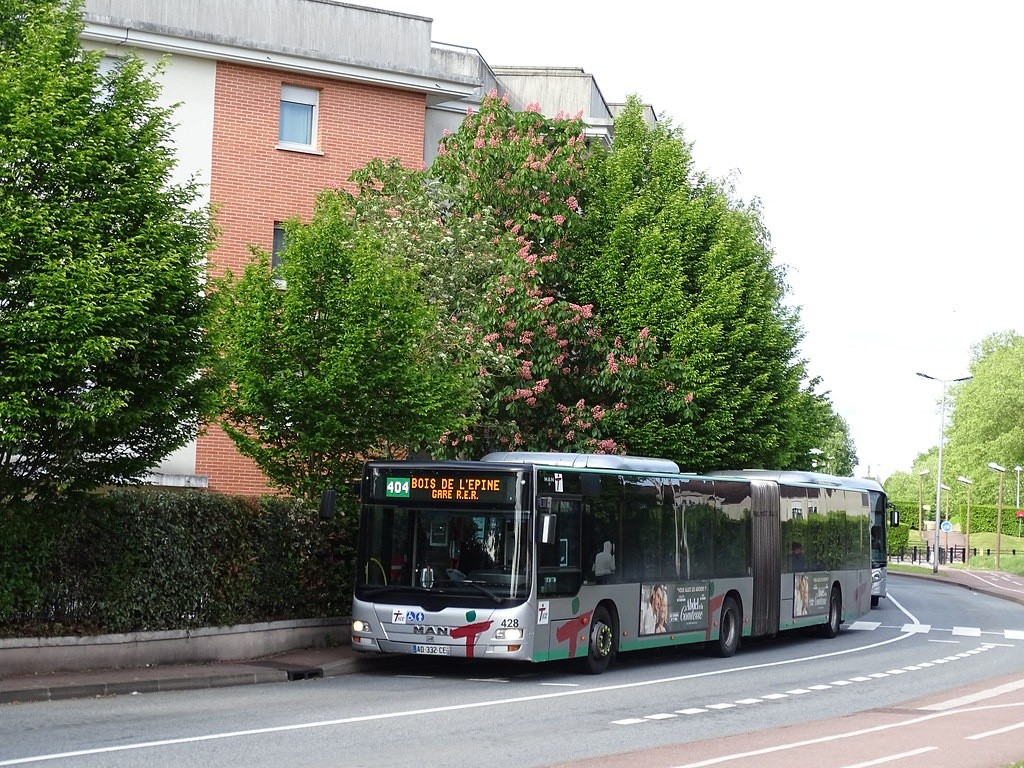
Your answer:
[641,585,667,634]
[795,575,808,615]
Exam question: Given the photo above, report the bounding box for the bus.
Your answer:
[320,452,870,675]
[837,477,899,607]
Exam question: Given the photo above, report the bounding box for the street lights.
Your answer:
[958,476,972,567]
[941,483,951,557]
[988,462,1006,568]
[918,469,930,541]
[916,370,974,575]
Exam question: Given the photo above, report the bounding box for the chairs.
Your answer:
[541,543,560,570]
